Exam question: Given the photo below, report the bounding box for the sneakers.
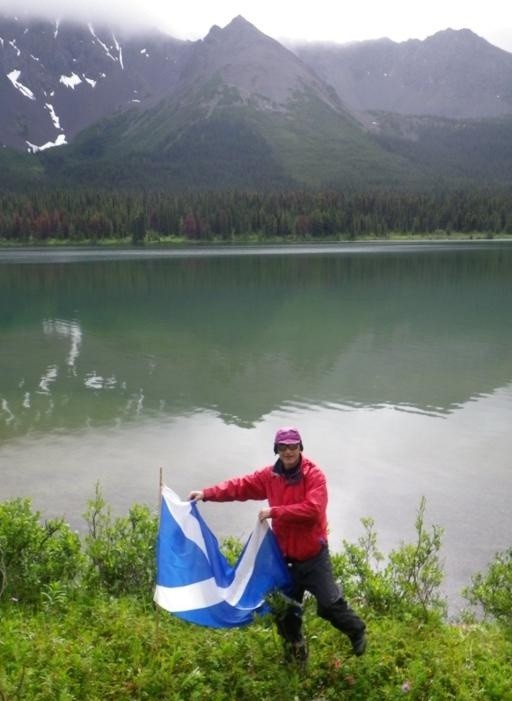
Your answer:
[352,635,366,655]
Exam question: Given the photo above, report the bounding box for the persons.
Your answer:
[187,427,368,667]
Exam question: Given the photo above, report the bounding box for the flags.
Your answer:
[152,484,294,631]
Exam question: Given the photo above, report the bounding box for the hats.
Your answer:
[273,427,304,455]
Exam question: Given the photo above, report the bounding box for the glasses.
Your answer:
[278,444,298,452]
